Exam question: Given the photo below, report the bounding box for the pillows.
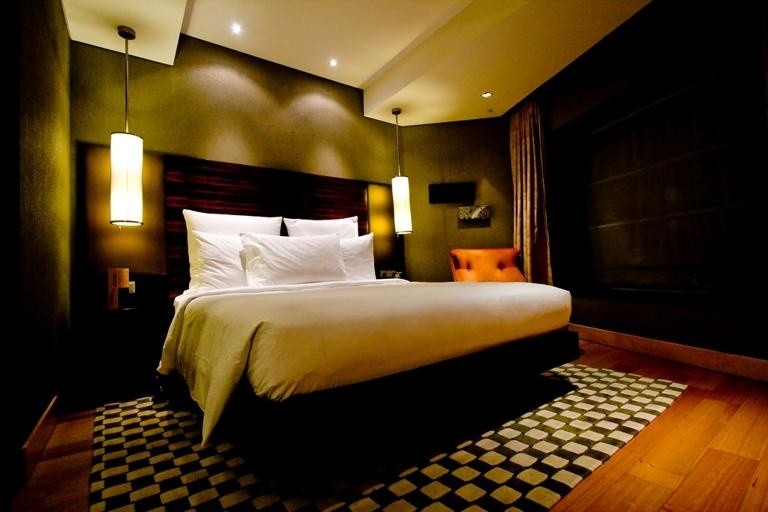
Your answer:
[183,210,380,290]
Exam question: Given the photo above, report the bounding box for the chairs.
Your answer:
[447,246,530,282]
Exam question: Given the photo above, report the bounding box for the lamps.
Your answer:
[391,108,413,239]
[106,23,146,229]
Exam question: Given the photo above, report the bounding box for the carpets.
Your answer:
[88,363,691,512]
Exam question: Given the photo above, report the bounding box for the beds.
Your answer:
[165,278,581,512]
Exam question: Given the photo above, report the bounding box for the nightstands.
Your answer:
[92,292,170,391]
